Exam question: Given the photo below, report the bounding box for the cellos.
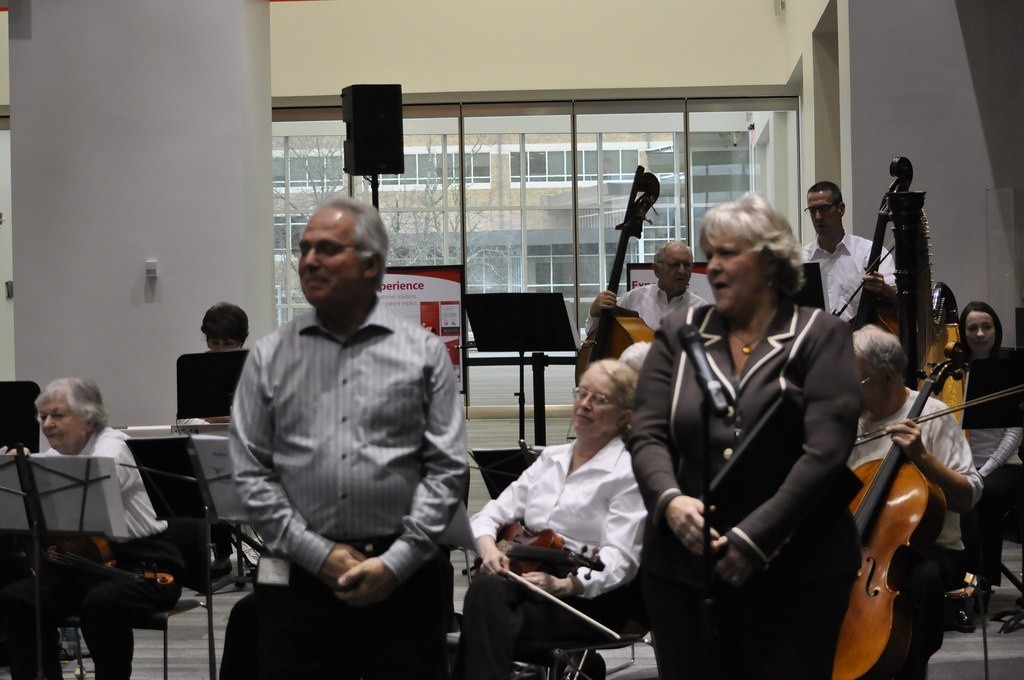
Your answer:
[828,337,972,680]
[574,164,660,387]
[840,153,915,383]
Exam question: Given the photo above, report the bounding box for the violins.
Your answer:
[475,520,606,580]
[22,534,176,592]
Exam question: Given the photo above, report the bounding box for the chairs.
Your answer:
[524,557,651,680]
[73,502,216,680]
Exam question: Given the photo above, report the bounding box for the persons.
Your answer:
[845,324,984,680]
[584,242,707,373]
[201,302,251,579]
[800,181,899,320]
[0,376,186,680]
[946,300,1024,632]
[452,354,651,680]
[626,192,862,680]
[227,197,469,680]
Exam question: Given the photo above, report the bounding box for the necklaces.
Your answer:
[734,332,763,354]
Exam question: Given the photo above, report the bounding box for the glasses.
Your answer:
[36,410,72,423]
[658,261,696,269]
[861,376,871,390]
[805,204,838,216]
[573,387,620,409]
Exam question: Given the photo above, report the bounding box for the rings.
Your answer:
[872,282,874,285]
[872,287,874,291]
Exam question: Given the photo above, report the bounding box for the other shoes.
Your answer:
[209,560,232,580]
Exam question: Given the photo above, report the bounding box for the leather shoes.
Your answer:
[955,612,977,633]
